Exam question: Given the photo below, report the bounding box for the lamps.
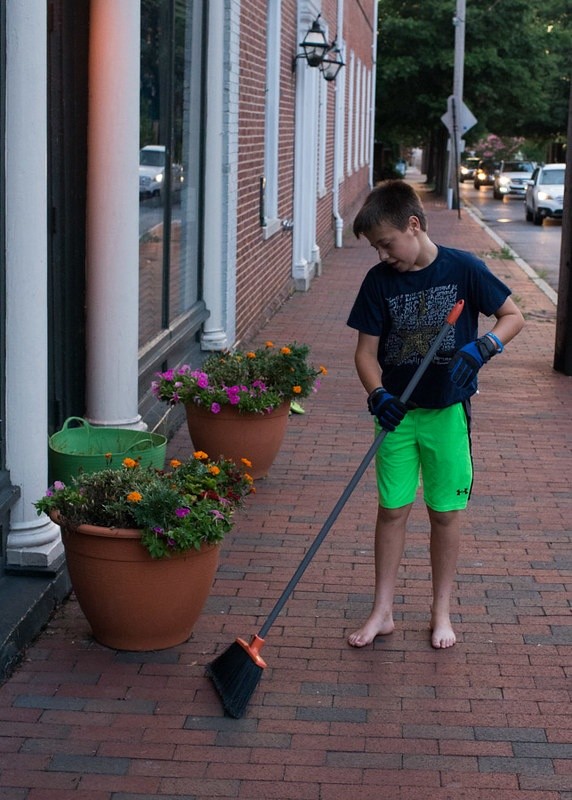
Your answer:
[291,12,329,73]
[322,36,346,82]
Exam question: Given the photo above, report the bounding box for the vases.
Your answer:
[187,395,292,478]
[45,511,220,652]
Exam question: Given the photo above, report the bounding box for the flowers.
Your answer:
[151,340,328,415]
[31,448,258,560]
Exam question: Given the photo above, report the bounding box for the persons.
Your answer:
[347,181,525,651]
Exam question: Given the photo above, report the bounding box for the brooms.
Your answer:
[201,298,468,720]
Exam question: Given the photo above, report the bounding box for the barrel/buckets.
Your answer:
[48,416,167,486]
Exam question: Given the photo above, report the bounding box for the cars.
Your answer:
[459,157,481,183]
[474,159,501,189]
[493,159,535,201]
[524,162,567,226]
[139,145,184,199]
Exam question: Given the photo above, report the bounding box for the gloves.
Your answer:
[366,387,406,432]
[447,336,498,389]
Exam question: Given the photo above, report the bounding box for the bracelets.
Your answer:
[486,332,504,353]
